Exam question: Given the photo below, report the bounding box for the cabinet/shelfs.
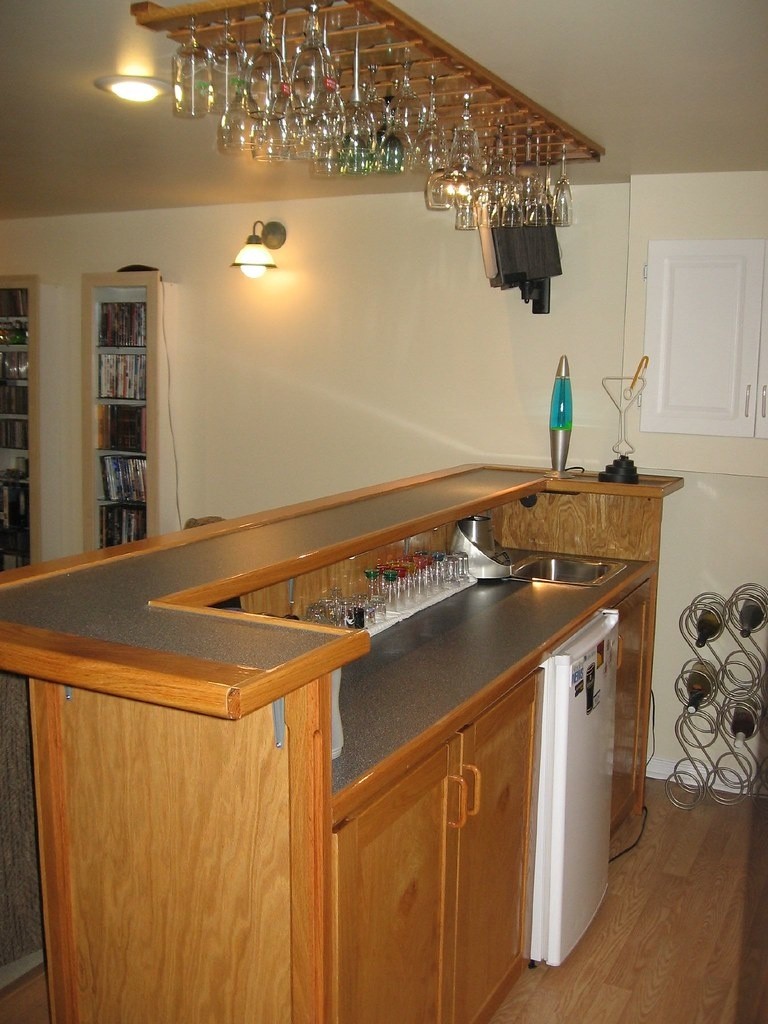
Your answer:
[638,240,768,439]
[0,274,42,572]
[81,272,148,548]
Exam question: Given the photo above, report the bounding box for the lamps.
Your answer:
[230,221,288,278]
[97,75,173,102]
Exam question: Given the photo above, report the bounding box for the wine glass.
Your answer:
[171,4,575,232]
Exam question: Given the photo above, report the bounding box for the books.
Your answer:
[98,303,146,547]
[0,290,28,570]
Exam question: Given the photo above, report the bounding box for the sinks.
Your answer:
[507,554,626,588]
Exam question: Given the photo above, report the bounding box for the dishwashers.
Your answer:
[531,609,619,966]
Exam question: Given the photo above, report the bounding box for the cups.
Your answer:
[304,546,470,631]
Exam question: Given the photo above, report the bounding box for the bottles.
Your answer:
[738,594,764,638]
[695,603,726,649]
[730,700,759,749]
[686,660,716,716]
